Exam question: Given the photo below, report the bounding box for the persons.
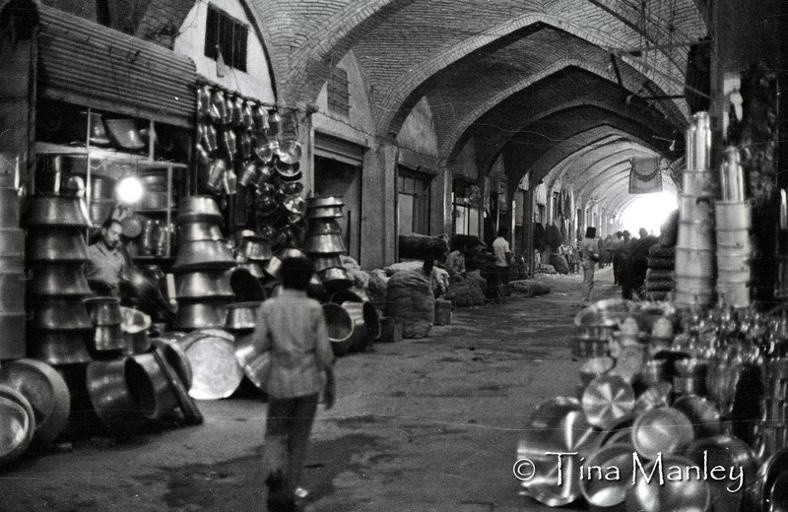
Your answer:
[86,217,126,296]
[581,226,648,305]
[249,256,338,512]
[492,226,513,296]
[445,242,468,281]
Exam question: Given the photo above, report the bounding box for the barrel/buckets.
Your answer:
[673,172,754,310]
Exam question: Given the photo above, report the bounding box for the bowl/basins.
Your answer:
[0,154,202,465]
[517,298,787,512]
[175,86,381,403]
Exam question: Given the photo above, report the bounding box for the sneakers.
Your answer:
[266,470,296,512]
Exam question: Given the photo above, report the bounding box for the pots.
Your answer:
[676,168,753,308]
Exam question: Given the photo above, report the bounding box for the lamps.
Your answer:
[483,209,487,218]
[669,137,676,152]
